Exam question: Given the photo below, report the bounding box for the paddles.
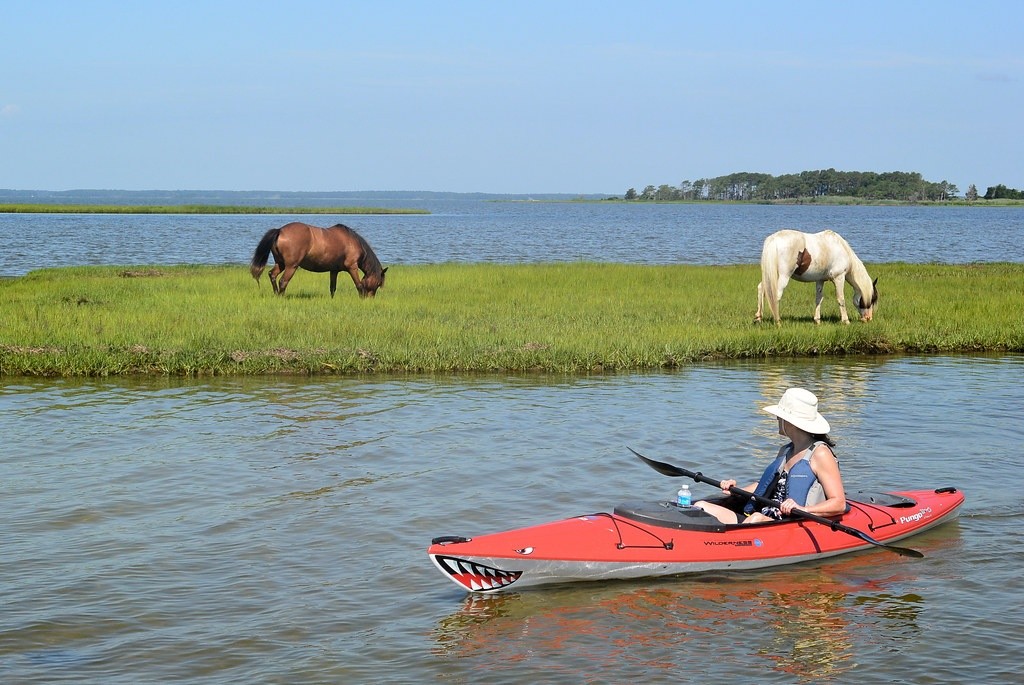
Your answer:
[625,444,926,561]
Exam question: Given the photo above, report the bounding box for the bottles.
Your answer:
[677,485,691,507]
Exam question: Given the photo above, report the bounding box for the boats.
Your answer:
[428,486,965,594]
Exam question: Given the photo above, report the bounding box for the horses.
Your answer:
[249,222,389,300]
[754,230,879,326]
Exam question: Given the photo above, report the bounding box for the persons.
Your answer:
[693,387,847,525]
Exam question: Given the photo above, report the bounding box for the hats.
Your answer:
[763,387,831,433]
